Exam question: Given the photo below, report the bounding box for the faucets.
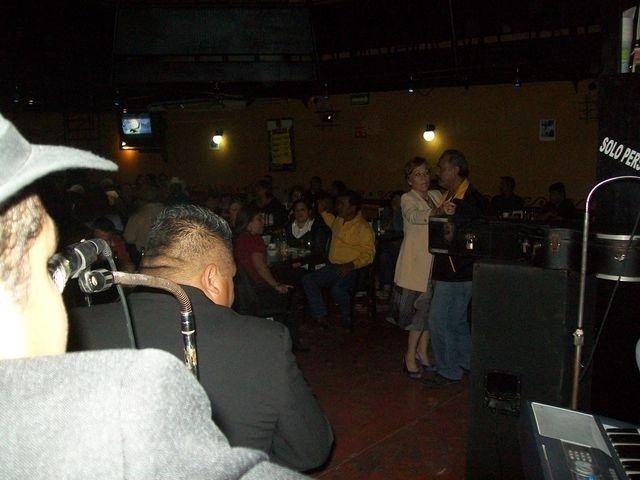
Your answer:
[521,239,531,253]
[532,240,543,256]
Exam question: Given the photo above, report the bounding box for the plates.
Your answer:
[288,247,297,252]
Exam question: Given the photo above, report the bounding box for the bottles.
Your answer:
[628,39,640,74]
[262,212,270,249]
[267,244,279,258]
[280,229,289,257]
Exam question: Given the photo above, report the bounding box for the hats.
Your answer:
[0,115,118,203]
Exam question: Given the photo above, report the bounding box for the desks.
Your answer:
[471,259,594,411]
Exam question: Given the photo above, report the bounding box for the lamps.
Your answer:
[212,127,224,146]
[422,124,438,143]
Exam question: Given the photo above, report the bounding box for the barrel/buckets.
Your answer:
[596,234,640,282]
[518,223,546,242]
[541,229,583,269]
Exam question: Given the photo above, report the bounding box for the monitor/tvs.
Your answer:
[119,110,168,146]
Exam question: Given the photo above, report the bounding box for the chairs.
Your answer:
[320,265,377,321]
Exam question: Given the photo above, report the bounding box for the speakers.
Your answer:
[467,262,597,480]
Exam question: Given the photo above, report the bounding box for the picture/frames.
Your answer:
[539,119,557,141]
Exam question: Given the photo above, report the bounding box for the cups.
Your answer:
[519,239,530,253]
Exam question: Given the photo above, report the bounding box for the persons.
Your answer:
[67,204,336,468]
[490,175,521,209]
[393,158,435,379]
[538,181,573,222]
[0,117,318,480]
[430,148,492,382]
[203,171,403,351]
[55,170,181,305]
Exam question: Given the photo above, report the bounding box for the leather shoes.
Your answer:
[403,350,470,386]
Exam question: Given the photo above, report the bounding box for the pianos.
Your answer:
[515,398,640,479]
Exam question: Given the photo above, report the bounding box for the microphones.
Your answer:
[47,237,106,297]
